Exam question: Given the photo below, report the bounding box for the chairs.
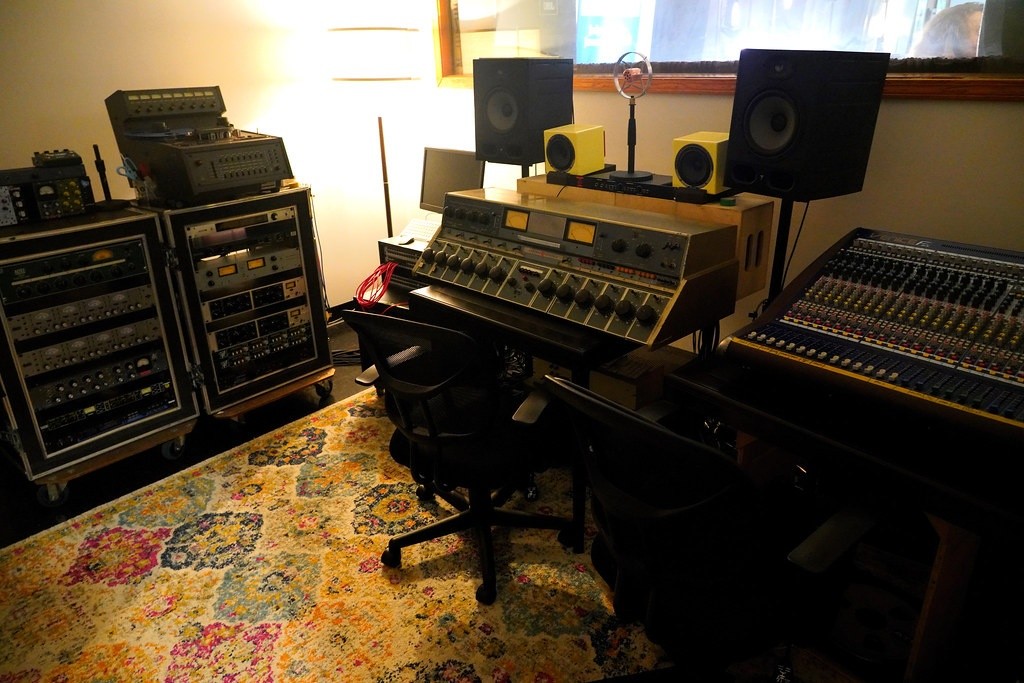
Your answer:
[342,306,580,605]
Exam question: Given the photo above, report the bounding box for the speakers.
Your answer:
[671,130,731,195]
[473,57,573,166]
[724,49,891,202]
[544,123,605,175]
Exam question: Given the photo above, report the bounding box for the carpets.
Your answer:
[1,384,1024,683]
